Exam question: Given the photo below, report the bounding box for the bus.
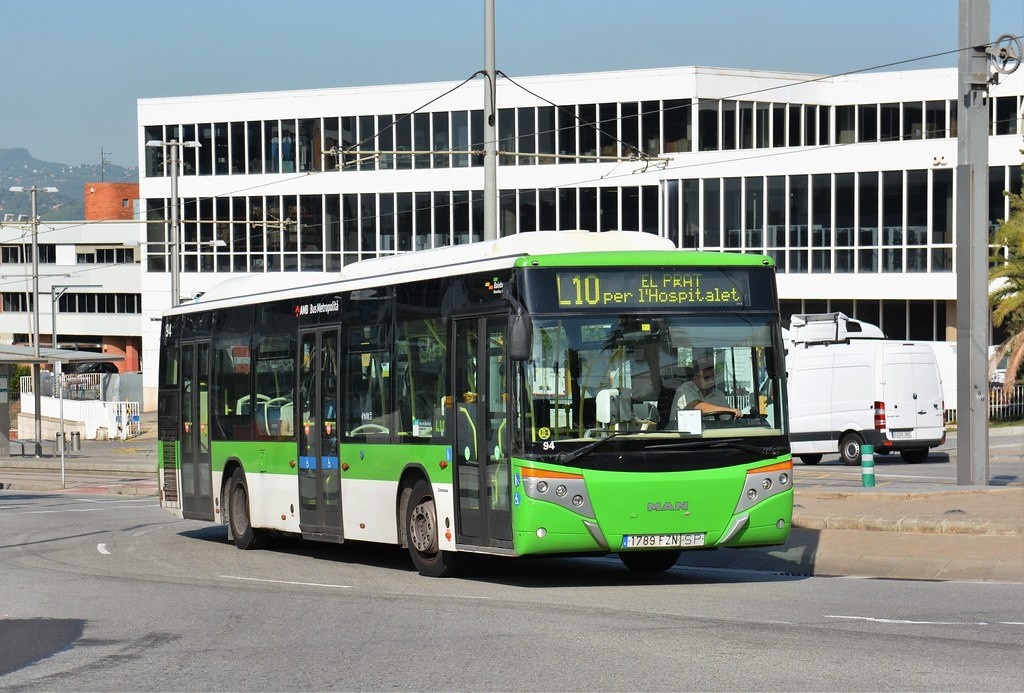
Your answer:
[157,231,794,579]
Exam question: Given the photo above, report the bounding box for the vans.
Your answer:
[739,312,947,466]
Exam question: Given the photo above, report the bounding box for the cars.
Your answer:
[72,362,119,385]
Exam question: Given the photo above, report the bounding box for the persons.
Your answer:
[279,376,336,438]
[656,355,743,430]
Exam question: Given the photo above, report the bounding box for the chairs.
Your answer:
[657,377,683,431]
[237,393,294,438]
[350,424,390,437]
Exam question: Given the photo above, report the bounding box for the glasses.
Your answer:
[704,376,714,382]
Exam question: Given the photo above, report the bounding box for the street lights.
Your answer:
[122,226,227,306]
[147,139,202,305]
[8,184,59,459]
